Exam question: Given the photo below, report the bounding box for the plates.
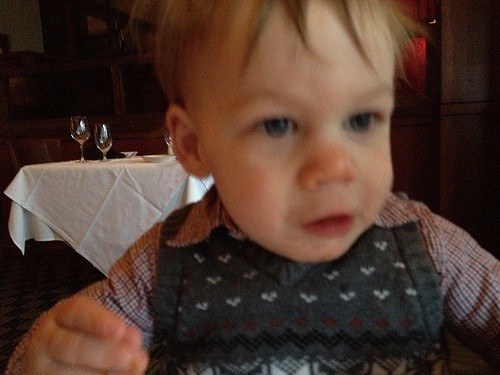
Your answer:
[142,155,178,163]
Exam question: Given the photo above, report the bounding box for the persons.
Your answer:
[4,0,500,375]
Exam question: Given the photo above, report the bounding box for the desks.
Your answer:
[21,156,213,276]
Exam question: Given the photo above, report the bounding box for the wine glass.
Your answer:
[70,114,91,165]
[93,122,114,162]
